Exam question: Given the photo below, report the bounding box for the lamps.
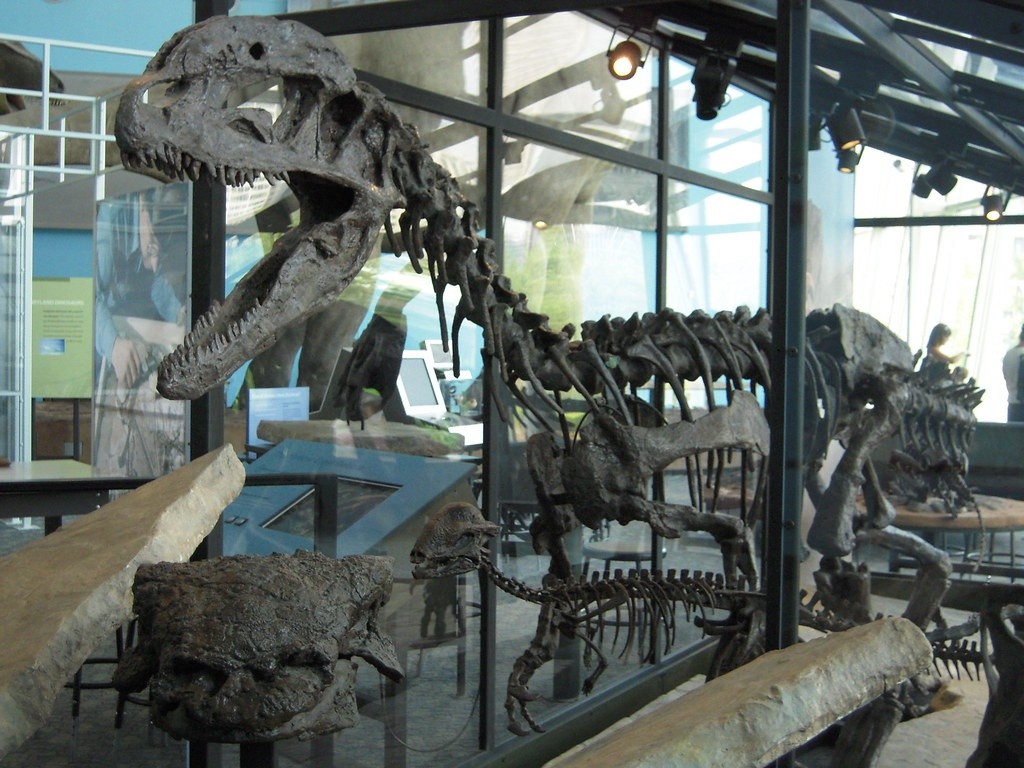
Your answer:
[912,131,969,199]
[605,8,659,80]
[690,32,743,120]
[808,89,869,173]
[980,182,1015,221]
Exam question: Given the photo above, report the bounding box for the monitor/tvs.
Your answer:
[421,340,455,372]
[383,350,446,421]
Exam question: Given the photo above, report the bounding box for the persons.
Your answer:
[97,183,191,389]
[1001,322,1024,422]
[928,323,965,365]
